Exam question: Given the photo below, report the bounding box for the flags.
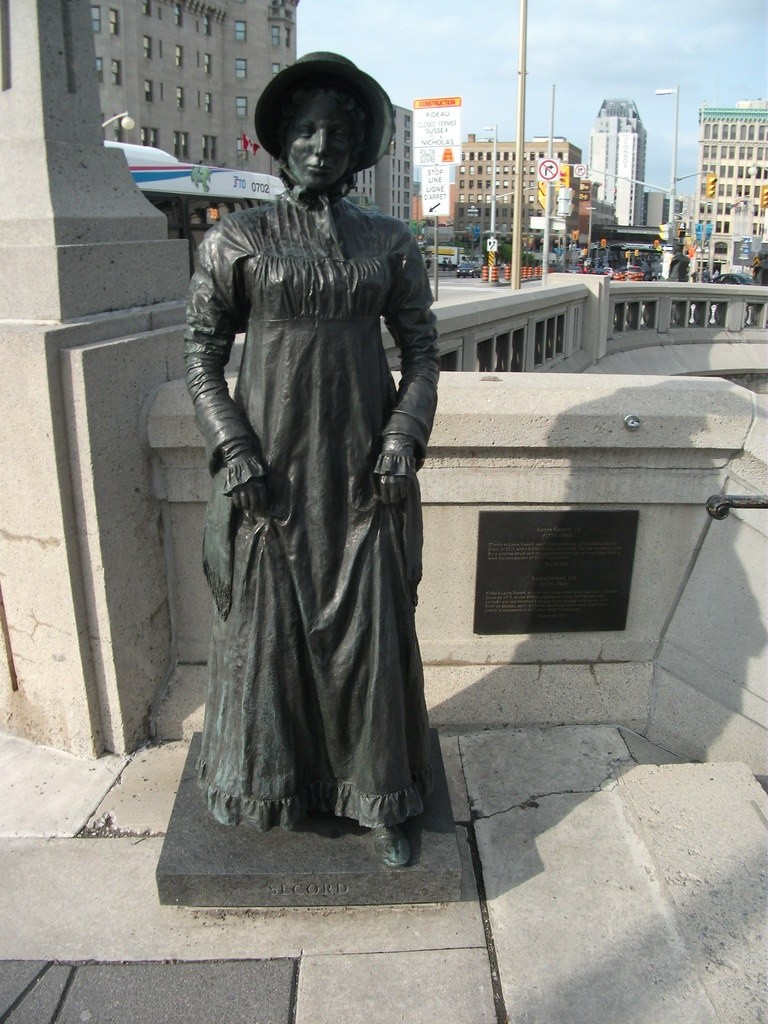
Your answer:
[241,132,260,156]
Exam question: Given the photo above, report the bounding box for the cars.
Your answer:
[568,263,660,281]
[456,261,481,278]
[713,272,752,285]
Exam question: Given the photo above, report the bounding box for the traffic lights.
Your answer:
[761,185,768,208]
[706,174,717,199]
[555,165,570,192]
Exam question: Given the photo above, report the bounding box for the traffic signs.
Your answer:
[421,165,450,218]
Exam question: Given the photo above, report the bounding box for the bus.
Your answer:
[104,140,284,281]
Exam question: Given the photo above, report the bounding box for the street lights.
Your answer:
[655,85,679,224]
[483,124,497,283]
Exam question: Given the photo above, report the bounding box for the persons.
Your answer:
[424,255,450,272]
[642,260,658,281]
[752,250,768,285]
[691,267,720,282]
[668,243,690,281]
[181,50,440,867]
